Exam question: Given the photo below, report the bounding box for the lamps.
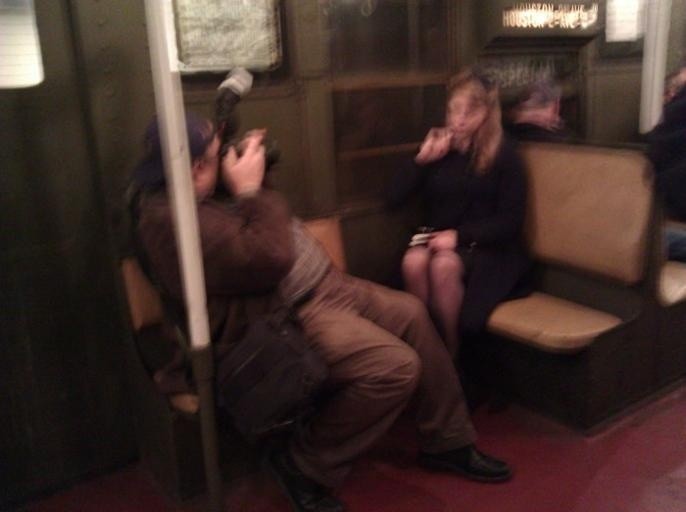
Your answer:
[504,2,599,31]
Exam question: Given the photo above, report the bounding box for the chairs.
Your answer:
[652,142,686,396]
[486,140,658,430]
[121,214,347,506]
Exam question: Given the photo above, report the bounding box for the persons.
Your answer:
[633,66,685,266]
[509,81,579,141]
[387,69,538,352]
[128,107,512,512]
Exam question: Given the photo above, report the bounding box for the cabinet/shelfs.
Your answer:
[303,70,450,277]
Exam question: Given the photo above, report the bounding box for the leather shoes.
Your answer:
[417,444,513,484]
[271,461,351,509]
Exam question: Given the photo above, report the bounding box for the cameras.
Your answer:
[207,65,282,184]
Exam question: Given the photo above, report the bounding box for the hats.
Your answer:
[128,118,213,187]
